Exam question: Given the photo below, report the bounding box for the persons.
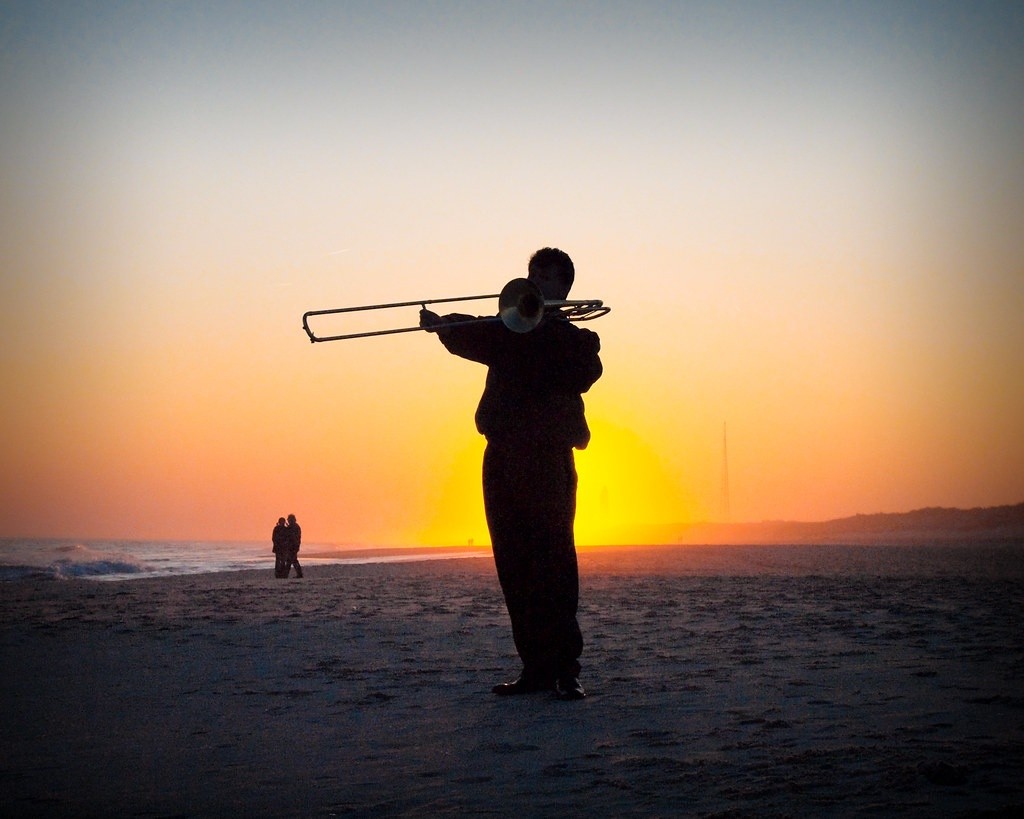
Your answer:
[280,513,304,579]
[421,246,603,700]
[272,517,288,579]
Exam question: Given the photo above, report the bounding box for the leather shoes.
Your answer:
[554,676,586,700]
[491,673,554,696]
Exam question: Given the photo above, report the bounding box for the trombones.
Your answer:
[300,278,612,342]
